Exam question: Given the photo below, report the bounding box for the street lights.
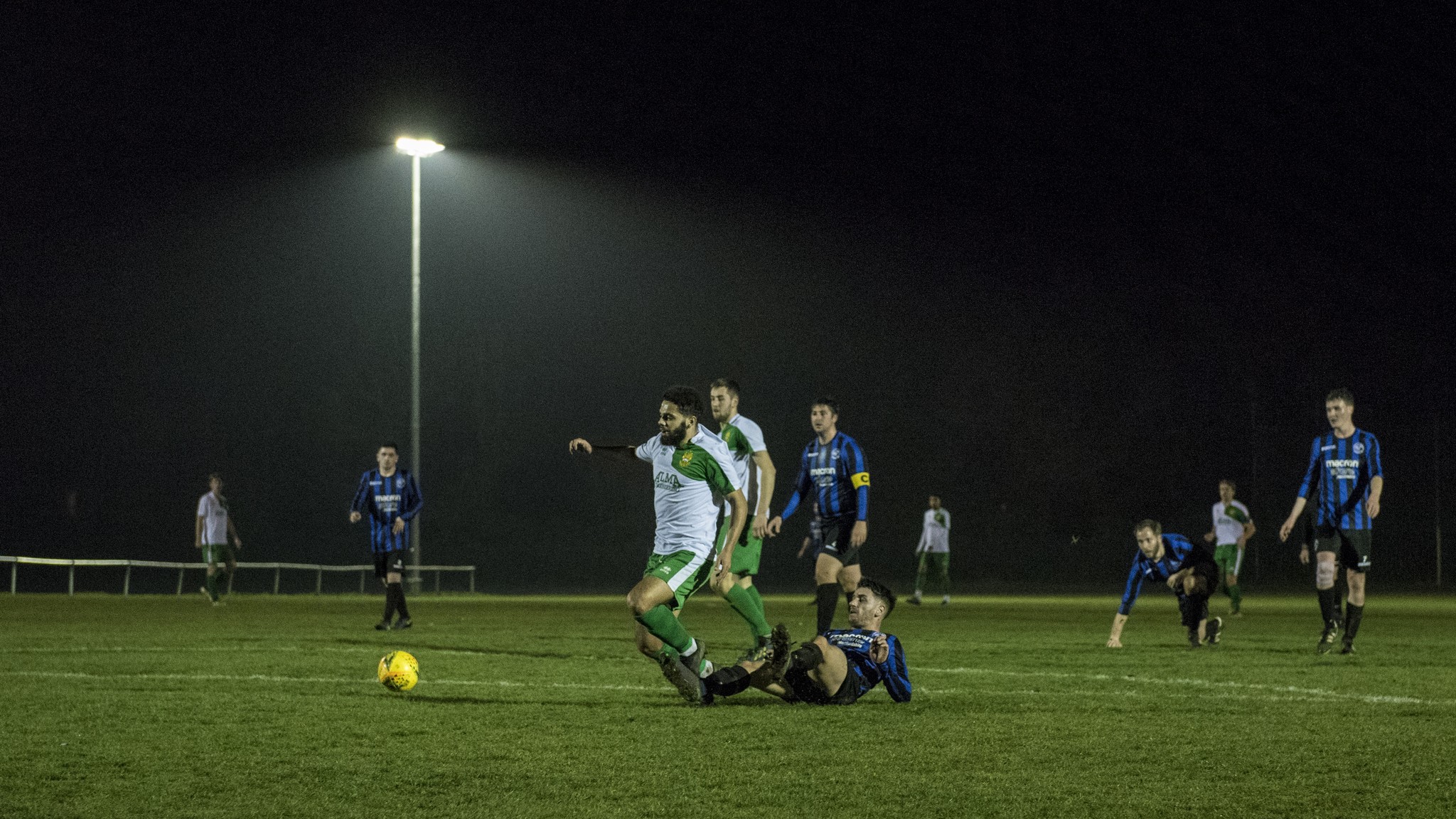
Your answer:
[390,134,450,596]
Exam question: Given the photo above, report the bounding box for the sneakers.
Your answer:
[662,639,715,679]
[1207,616,1222,646]
[201,585,220,607]
[659,656,712,706]
[1316,625,1339,654]
[770,624,792,683]
[906,597,921,606]
[376,618,391,630]
[1341,647,1358,658]
[392,619,412,630]
[747,636,774,662]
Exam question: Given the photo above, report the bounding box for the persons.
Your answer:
[762,396,872,640]
[1299,473,1346,635]
[349,440,422,633]
[1279,389,1386,657]
[707,375,776,651]
[1104,518,1222,649]
[906,491,951,610]
[656,578,912,705]
[1202,479,1257,617]
[568,386,750,702]
[194,470,246,609]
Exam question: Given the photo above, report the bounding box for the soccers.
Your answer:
[378,650,419,692]
